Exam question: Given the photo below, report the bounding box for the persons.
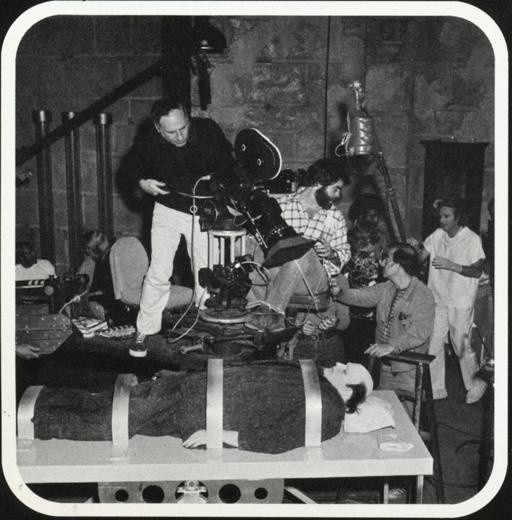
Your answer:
[336,173,386,232]
[15,345,41,362]
[114,93,233,358]
[404,199,489,404]
[17,360,374,456]
[16,241,58,304]
[347,194,388,321]
[226,157,353,332]
[329,241,436,422]
[78,229,115,321]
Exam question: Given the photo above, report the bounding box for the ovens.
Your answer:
[16,380,434,503]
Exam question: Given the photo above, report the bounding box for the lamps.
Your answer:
[109,235,195,311]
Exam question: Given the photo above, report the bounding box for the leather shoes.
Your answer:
[431,256,463,273]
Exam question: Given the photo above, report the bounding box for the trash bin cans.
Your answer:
[327,247,338,262]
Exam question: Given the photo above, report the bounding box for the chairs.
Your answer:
[247,302,277,314]
[466,380,487,404]
[128,332,148,358]
[245,316,286,332]
[432,389,448,401]
